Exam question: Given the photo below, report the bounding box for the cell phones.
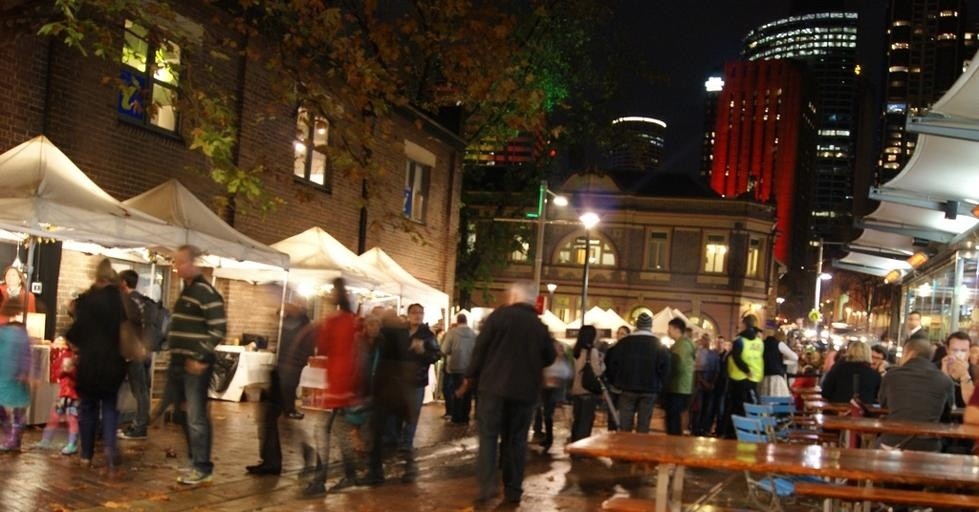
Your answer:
[956,350,965,361]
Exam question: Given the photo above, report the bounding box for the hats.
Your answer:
[636,313,651,328]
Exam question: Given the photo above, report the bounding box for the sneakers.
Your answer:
[1,424,146,468]
[179,469,210,486]
[285,409,304,421]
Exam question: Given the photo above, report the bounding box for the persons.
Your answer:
[457,279,555,508]
[0,244,443,498]
[440,309,799,467]
[791,310,978,498]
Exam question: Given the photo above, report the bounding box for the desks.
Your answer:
[562,385,979,511]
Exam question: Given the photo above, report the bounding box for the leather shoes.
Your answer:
[245,453,409,498]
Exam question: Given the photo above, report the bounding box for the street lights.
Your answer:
[543,277,559,313]
[576,208,600,326]
[812,269,834,334]
[530,178,571,300]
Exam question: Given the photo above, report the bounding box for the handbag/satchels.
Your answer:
[581,362,602,396]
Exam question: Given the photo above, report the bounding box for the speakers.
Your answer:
[945,199,959,220]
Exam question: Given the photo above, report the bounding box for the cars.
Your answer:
[786,328,829,353]
